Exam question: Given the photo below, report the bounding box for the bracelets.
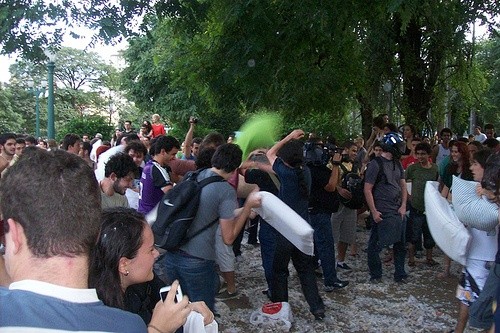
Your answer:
[148,324,163,333]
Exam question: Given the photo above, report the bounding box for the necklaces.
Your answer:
[100,180,105,193]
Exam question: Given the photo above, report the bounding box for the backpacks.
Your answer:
[152,174,227,250]
[337,163,364,209]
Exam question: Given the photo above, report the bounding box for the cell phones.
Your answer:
[159,284,181,305]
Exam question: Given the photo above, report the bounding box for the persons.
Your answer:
[88,207,214,333]
[304,114,500,333]
[0,145,148,333]
[0,114,327,322]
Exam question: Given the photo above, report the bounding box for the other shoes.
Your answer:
[425,258,440,266]
[315,313,324,319]
[215,289,238,300]
[408,259,414,266]
[326,280,350,293]
[337,262,352,272]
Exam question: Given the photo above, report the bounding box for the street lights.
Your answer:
[34,77,48,140]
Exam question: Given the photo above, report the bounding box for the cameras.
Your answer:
[194,118,198,123]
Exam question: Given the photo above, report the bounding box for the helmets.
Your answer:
[382,133,406,155]
